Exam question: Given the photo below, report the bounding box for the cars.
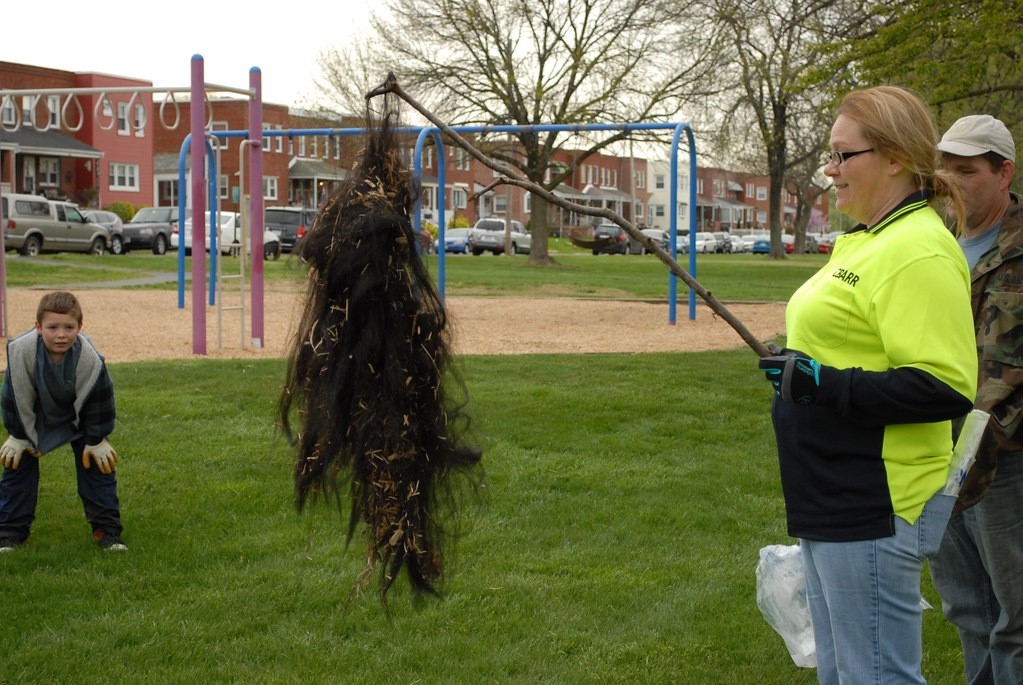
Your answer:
[730,236,743,253]
[434,228,475,255]
[676,236,686,254]
[741,235,759,251]
[121,207,188,254]
[169,210,283,261]
[782,230,846,253]
[751,239,771,255]
[80,210,126,254]
[685,232,718,255]
[640,229,671,253]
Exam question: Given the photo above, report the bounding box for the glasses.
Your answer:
[826,148,874,166]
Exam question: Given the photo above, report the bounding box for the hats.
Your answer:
[936,114,1016,163]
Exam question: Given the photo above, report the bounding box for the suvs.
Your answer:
[592,223,650,255]
[264,207,320,254]
[713,232,732,254]
[470,218,532,256]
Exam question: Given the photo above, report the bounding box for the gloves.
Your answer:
[758,343,823,407]
[0,434,41,470]
[82,438,118,475]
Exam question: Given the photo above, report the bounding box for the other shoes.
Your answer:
[0,541,15,552]
[97,533,129,551]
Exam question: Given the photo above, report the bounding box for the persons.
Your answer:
[0,291,127,551]
[758,85,978,685]
[927,114,1023,685]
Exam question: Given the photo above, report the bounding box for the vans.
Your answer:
[1,192,112,257]
